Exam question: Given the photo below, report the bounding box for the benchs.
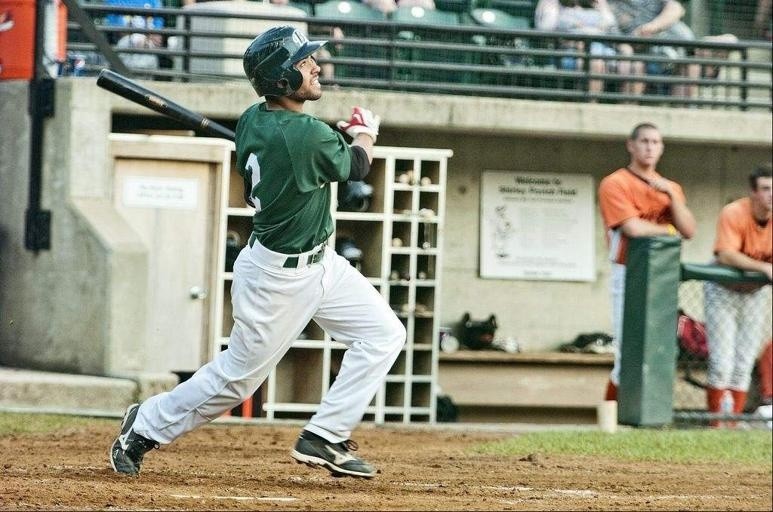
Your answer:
[438,349,707,411]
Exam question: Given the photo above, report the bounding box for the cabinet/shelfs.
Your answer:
[210,142,454,425]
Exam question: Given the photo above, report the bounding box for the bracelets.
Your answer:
[665,220,676,235]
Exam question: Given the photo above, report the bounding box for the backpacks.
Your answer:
[676,309,726,391]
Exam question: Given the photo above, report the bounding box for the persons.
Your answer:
[535,0,643,104]
[359,0,435,20]
[96,0,164,70]
[702,161,773,427]
[269,0,344,90]
[609,0,739,107]
[595,124,697,427]
[109,21,409,482]
[755,333,773,417]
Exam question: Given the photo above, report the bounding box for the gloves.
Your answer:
[336,106,382,143]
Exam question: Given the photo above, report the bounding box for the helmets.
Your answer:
[340,178,374,212]
[225,228,246,268]
[242,25,328,99]
[334,236,364,273]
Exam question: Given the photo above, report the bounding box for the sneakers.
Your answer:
[109,402,160,475]
[562,333,615,355]
[291,432,374,479]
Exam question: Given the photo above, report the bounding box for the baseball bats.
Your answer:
[96,69,235,141]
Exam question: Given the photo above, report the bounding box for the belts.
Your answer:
[248,234,326,270]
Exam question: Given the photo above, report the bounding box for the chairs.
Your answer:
[288,2,535,91]
